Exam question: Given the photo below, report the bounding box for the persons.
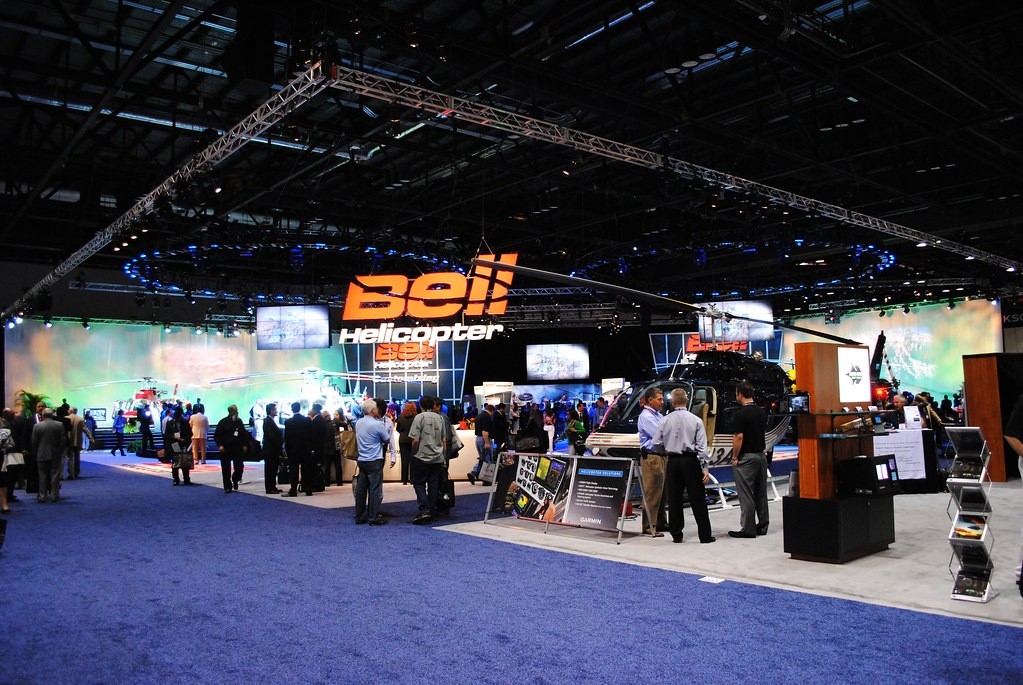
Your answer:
[82,409,97,451]
[308,404,350,495]
[111,410,128,457]
[886,392,965,429]
[353,397,400,526]
[189,408,209,463]
[1004,435,1023,600]
[0,408,26,511]
[63,407,95,478]
[61,398,70,414]
[282,402,313,497]
[138,404,154,451]
[159,398,204,461]
[395,395,459,525]
[637,387,717,543]
[553,397,610,454]
[213,405,249,494]
[27,402,67,503]
[163,407,197,486]
[727,383,770,539]
[467,401,556,487]
[260,404,284,494]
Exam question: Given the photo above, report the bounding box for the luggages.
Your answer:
[507,427,516,450]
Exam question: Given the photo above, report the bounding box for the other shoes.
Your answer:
[369,517,389,526]
[646,529,663,537]
[233,479,239,490]
[730,531,754,538]
[266,480,343,497]
[468,473,474,484]
[700,537,716,543]
[224,488,231,493]
[413,511,433,523]
[483,481,491,486]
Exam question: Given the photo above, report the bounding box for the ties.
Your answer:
[580,413,583,421]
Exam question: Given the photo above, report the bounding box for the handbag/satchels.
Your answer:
[172,453,194,469]
[340,431,361,460]
[451,436,464,453]
[574,433,587,447]
[112,418,119,433]
[478,448,495,483]
[2,446,27,475]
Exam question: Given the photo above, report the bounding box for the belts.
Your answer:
[740,448,763,454]
[645,451,667,456]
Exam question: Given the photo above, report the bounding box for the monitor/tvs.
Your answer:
[257,305,330,349]
[526,344,590,379]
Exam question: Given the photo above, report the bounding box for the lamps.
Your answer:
[0,0,1023,339]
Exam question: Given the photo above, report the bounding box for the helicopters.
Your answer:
[211,368,382,449]
[79,376,210,437]
[472,257,863,509]
